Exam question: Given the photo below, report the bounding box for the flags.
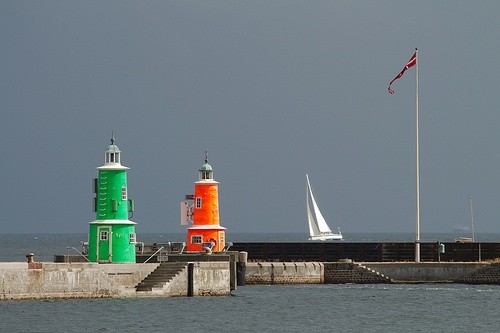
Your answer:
[387,50,416,94]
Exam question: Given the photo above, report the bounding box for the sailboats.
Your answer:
[305,174,343,240]
